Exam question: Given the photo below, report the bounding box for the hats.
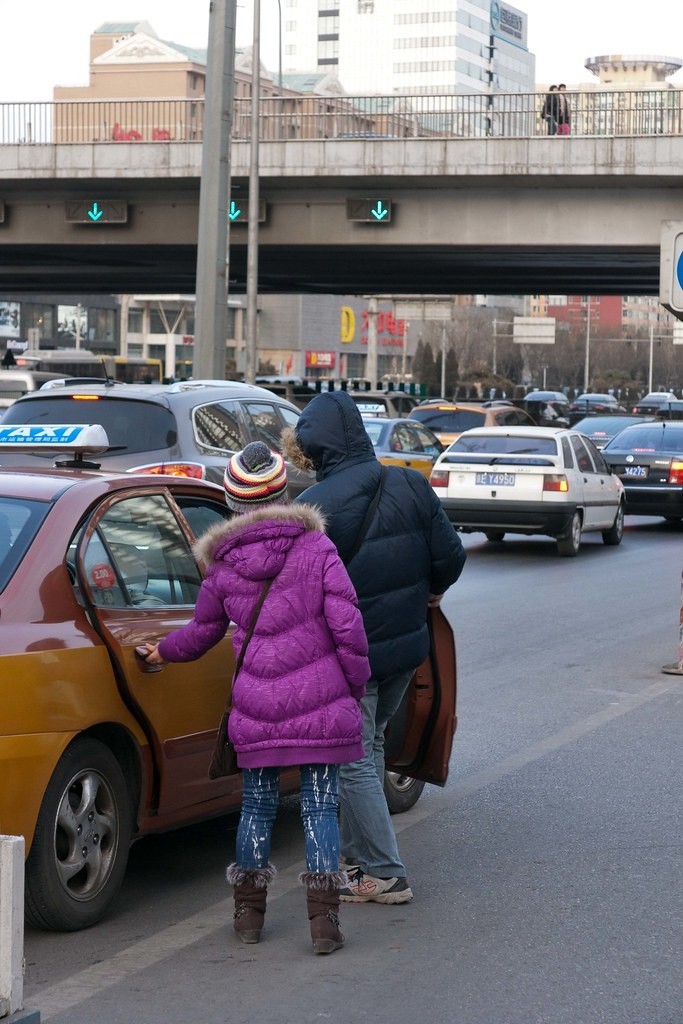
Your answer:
[223,441,289,513]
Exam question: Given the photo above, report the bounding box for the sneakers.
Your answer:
[338,856,359,876]
[338,866,413,904]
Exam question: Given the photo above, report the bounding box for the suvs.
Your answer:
[349,391,420,432]
[393,398,540,452]
[0,377,316,506]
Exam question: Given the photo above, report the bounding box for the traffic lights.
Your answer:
[226,201,267,223]
[345,198,391,223]
[64,199,128,224]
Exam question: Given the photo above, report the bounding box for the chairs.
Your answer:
[85,541,166,604]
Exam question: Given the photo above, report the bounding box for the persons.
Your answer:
[541,84,570,136]
[144,446,373,957]
[291,392,469,904]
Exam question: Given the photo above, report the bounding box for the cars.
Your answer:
[360,416,447,484]
[524,390,571,416]
[430,424,626,556]
[0,369,72,423]
[257,382,320,412]
[483,399,570,429]
[568,413,666,461]
[241,377,302,386]
[602,419,683,527]
[0,422,459,937]
[655,400,683,422]
[633,391,679,418]
[568,393,627,423]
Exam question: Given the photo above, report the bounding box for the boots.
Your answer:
[299,870,345,953]
[227,861,277,944]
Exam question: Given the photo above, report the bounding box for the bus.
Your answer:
[382,374,413,386]
[0,348,193,384]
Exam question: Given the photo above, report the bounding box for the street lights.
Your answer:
[403,321,411,386]
[542,366,549,389]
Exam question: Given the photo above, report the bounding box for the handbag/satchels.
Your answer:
[209,704,243,779]
